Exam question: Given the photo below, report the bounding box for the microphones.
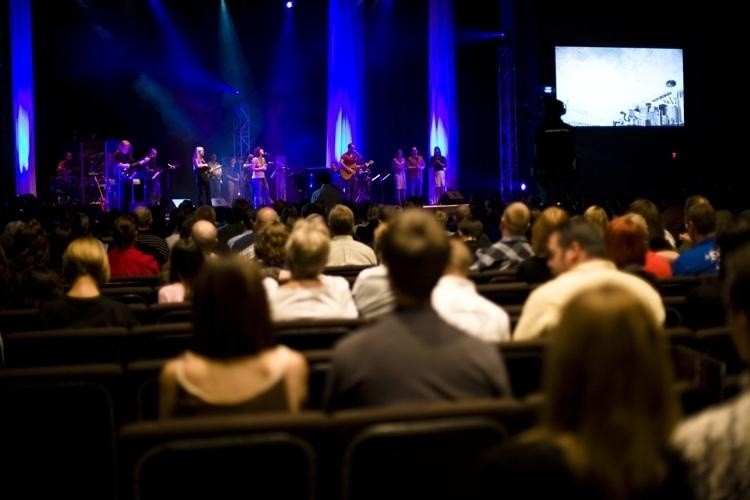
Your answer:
[353,150,360,156]
[265,152,271,156]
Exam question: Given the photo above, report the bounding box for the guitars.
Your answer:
[341,160,374,180]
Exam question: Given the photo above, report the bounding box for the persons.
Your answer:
[473,193,749,500]
[0,193,170,360]
[407,147,425,198]
[224,145,269,205]
[158,205,505,420]
[55,139,163,204]
[392,148,408,205]
[193,146,222,205]
[428,147,447,204]
[330,143,371,205]
